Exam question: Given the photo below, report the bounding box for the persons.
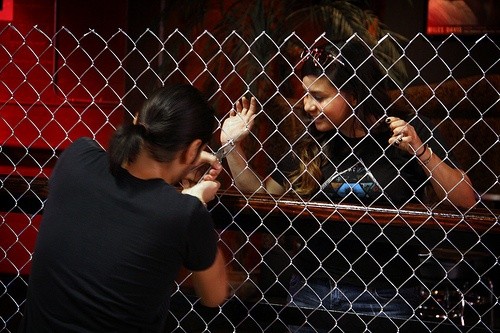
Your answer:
[29,85,228,333]
[220,41,475,333]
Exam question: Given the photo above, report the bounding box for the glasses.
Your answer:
[300,46,349,69]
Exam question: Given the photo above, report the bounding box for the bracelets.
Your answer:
[416,142,433,166]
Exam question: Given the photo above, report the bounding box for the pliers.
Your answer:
[190,139,236,183]
